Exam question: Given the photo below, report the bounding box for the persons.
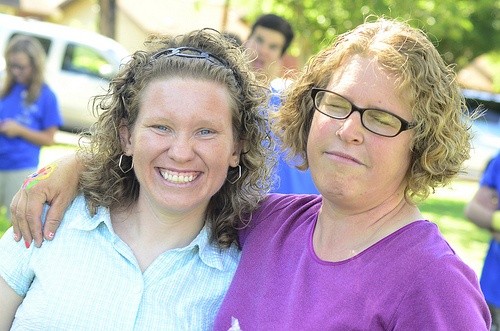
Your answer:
[0,27,278,331]
[0,29,63,212]
[465,151,500,331]
[8,14,493,331]
[242,15,318,195]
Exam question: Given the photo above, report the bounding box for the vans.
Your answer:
[2,14,130,135]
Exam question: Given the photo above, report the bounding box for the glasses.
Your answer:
[9,63,30,69]
[311,87,418,138]
